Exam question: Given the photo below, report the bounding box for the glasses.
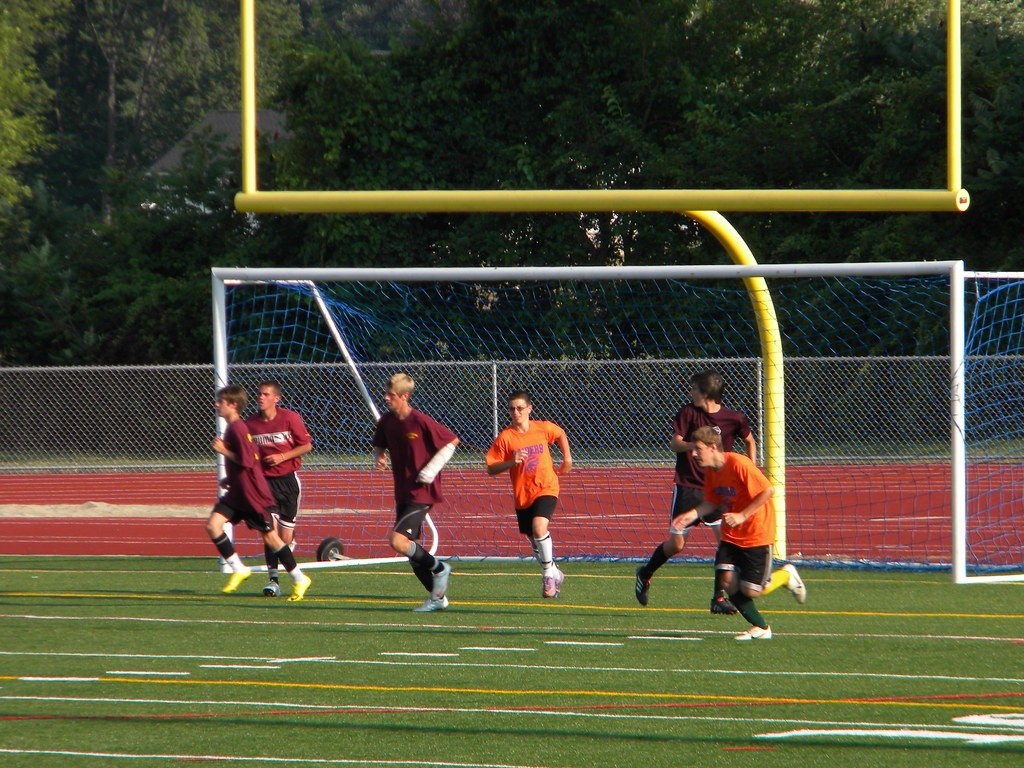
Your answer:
[509,406,528,412]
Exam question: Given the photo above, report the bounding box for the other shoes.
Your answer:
[263,582,281,598]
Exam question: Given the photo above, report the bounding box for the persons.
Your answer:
[671,426,806,641]
[372,374,460,613]
[206,385,311,602]
[246,381,311,595]
[635,370,756,614]
[485,390,572,598]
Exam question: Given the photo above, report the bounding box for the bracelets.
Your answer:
[281,453,285,461]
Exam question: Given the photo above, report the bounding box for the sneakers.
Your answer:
[733,626,772,640]
[431,561,451,602]
[287,575,311,601]
[555,571,564,596]
[542,575,555,597]
[221,568,250,593]
[635,566,651,606]
[413,595,448,612]
[710,598,737,614]
[782,563,805,604]
